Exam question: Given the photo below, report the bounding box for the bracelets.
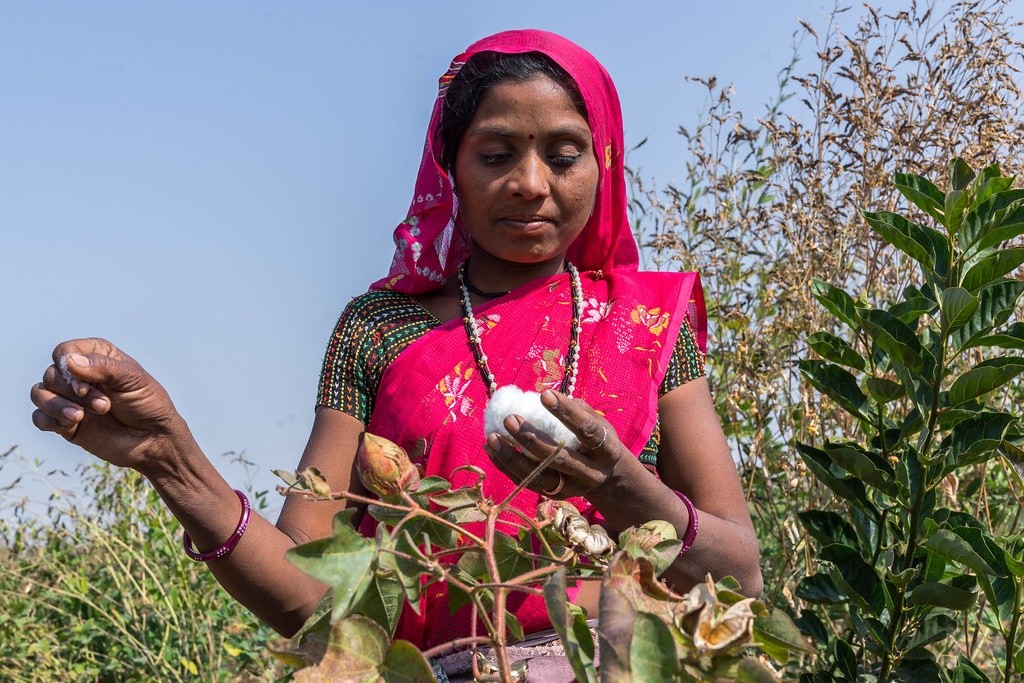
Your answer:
[672,489,699,558]
[183,489,251,562]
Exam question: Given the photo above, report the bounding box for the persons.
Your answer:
[31,29,765,683]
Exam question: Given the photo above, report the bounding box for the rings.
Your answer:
[584,427,607,450]
[543,471,564,496]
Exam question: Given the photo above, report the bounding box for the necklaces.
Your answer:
[456,255,584,401]
